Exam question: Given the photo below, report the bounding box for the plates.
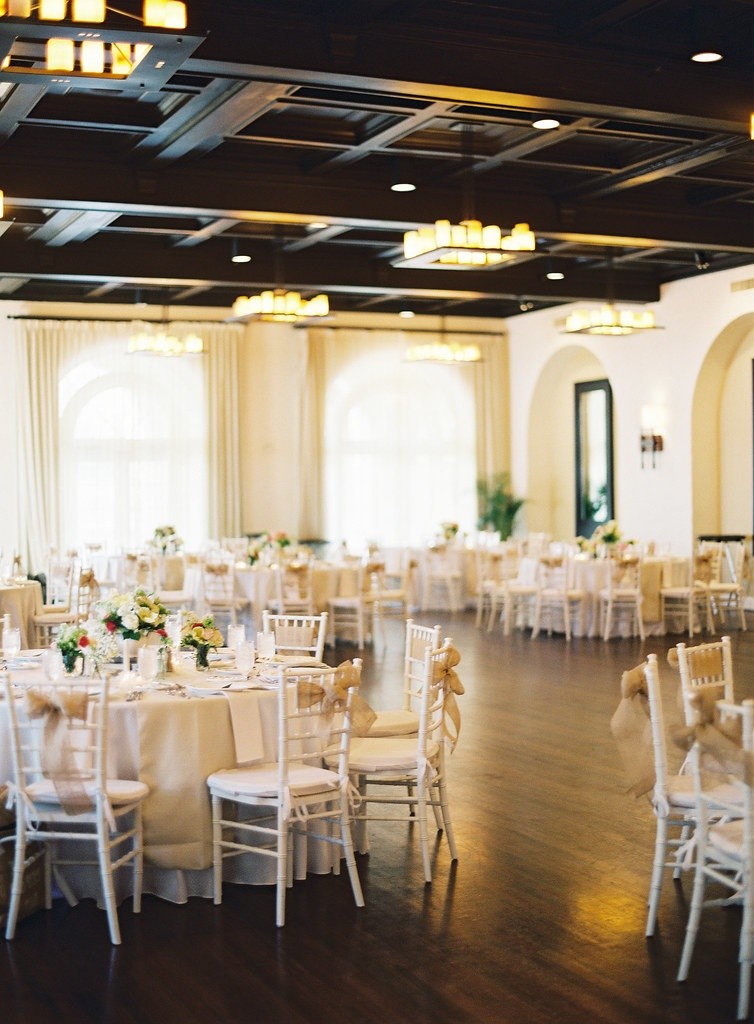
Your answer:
[7,661,40,670]
[210,653,234,661]
[284,655,317,664]
[216,668,241,676]
[260,672,280,683]
[177,679,232,696]
[153,681,173,690]
[15,649,47,660]
[16,580,39,584]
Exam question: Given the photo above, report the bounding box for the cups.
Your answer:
[7,578,15,586]
[258,631,274,658]
[227,624,245,651]
[3,627,21,663]
[171,652,195,675]
[132,663,137,672]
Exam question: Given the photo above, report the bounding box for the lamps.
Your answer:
[224,289,335,324]
[558,247,666,337]
[0,0,210,94]
[405,300,484,363]
[694,249,709,269]
[393,123,550,271]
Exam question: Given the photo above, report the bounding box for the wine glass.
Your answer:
[235,640,255,681]
[138,648,160,694]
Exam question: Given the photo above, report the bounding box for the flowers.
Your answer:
[146,525,183,553]
[101,587,169,640]
[54,621,119,676]
[178,610,224,667]
[441,521,459,544]
[573,518,640,559]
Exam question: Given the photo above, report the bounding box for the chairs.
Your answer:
[0,530,754,1021]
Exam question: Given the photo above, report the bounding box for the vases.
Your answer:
[195,647,210,671]
[91,659,105,681]
[124,633,160,673]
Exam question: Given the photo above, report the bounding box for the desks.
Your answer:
[0,575,49,650]
[0,647,370,911]
[500,555,689,639]
